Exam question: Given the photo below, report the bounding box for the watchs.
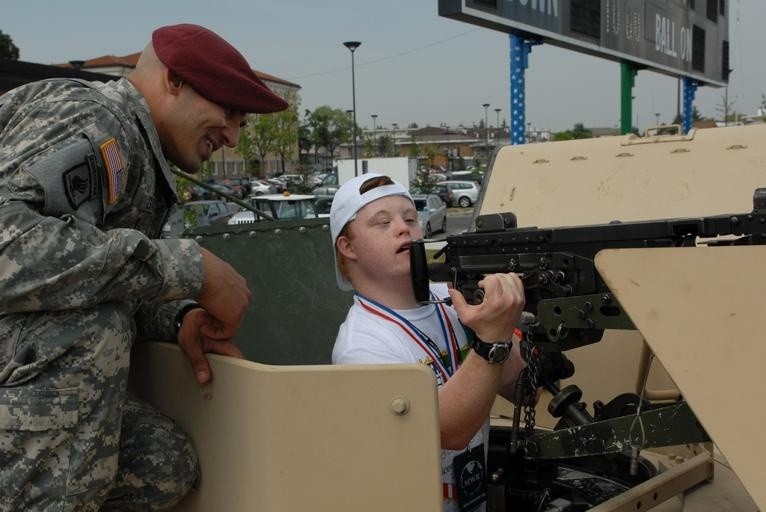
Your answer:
[472,333,513,366]
[174,303,204,340]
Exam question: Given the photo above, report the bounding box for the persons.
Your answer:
[328,172,541,512]
[0,22,287,512]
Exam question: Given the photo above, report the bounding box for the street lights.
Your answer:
[370,114,378,154]
[342,36,361,179]
[345,109,353,159]
[525,121,532,143]
[481,102,490,147]
[492,108,502,146]
[390,122,398,155]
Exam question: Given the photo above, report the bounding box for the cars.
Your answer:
[160,163,486,238]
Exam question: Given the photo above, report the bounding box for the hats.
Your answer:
[153,24,293,113]
[330,173,416,292]
